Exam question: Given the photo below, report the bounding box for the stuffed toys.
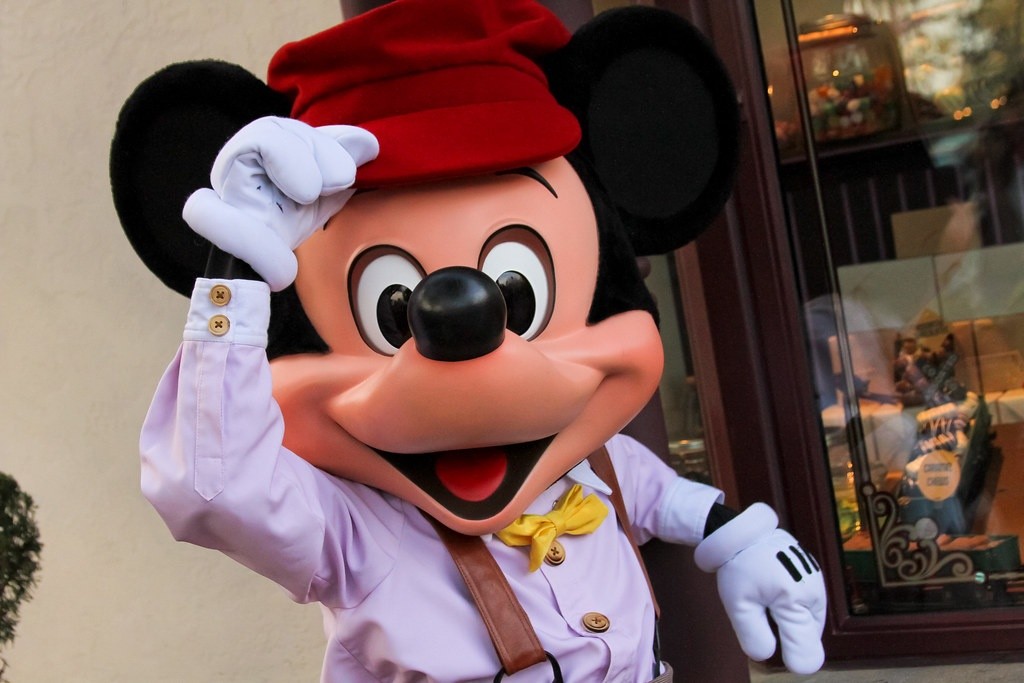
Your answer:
[109,0,829,683]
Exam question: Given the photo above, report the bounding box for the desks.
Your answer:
[780,91,1024,302]
